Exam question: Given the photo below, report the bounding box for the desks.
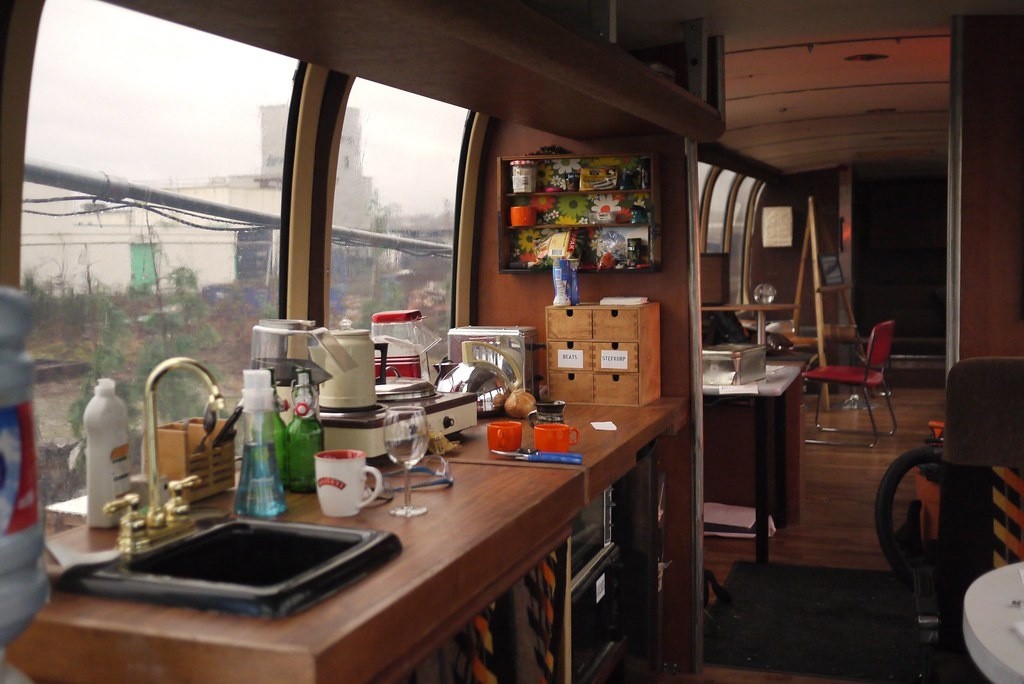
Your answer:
[702,358,804,564]
[963,560,1024,684]
[702,301,797,351]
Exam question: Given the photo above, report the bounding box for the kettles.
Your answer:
[309,316,389,408]
[433,342,522,418]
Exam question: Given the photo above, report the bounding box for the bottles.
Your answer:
[259,367,289,489]
[0,285,49,649]
[285,368,325,493]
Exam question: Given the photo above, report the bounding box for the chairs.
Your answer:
[801,319,898,449]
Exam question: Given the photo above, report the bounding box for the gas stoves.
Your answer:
[319,392,478,459]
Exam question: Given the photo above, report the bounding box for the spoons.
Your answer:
[193,401,217,453]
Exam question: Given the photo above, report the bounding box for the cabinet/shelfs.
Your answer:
[494,151,661,274]
[544,299,661,407]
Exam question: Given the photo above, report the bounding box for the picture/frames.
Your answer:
[819,252,844,285]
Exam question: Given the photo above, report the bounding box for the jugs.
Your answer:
[370,309,442,379]
[249,319,358,408]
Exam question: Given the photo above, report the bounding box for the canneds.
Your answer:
[509,161,536,193]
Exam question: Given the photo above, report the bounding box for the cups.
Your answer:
[534,424,580,453]
[314,450,382,518]
[487,421,522,452]
[527,400,567,429]
[566,174,579,191]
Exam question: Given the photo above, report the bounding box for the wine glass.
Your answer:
[384,406,429,517]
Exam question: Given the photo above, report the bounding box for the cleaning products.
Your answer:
[82,377,130,527]
[234,370,286,517]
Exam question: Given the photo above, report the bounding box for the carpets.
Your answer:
[698,557,935,684]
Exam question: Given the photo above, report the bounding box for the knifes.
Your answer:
[491,448,582,465]
[211,398,244,448]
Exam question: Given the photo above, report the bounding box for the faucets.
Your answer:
[145,356,226,527]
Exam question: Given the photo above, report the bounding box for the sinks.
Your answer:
[56,516,403,619]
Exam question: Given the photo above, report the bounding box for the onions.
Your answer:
[506,390,536,419]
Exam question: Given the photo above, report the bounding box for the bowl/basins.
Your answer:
[511,206,537,226]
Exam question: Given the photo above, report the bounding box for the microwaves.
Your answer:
[571,521,626,684]
